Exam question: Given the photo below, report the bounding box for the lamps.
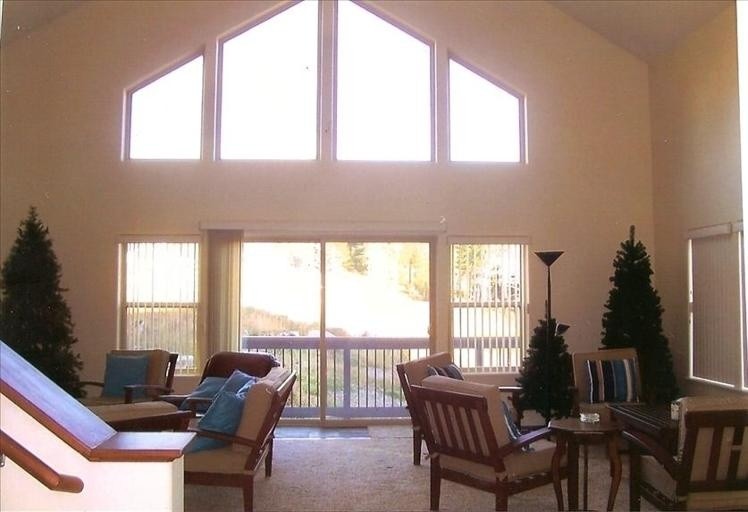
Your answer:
[534,251,570,337]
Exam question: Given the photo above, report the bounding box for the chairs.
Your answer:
[621,396,748,512]
[396,350,524,467]
[571,346,642,477]
[62,348,178,408]
[184,366,297,512]
[409,373,570,512]
[150,352,281,432]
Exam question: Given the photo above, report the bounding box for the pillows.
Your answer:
[178,376,228,413]
[101,351,148,397]
[236,376,256,393]
[584,358,637,403]
[654,419,679,442]
[183,392,245,455]
[212,368,252,398]
[446,363,464,380]
[434,366,446,375]
[502,400,528,453]
[427,365,438,376]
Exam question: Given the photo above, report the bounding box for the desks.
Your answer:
[84,400,193,433]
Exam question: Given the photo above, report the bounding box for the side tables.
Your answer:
[548,415,627,511]
